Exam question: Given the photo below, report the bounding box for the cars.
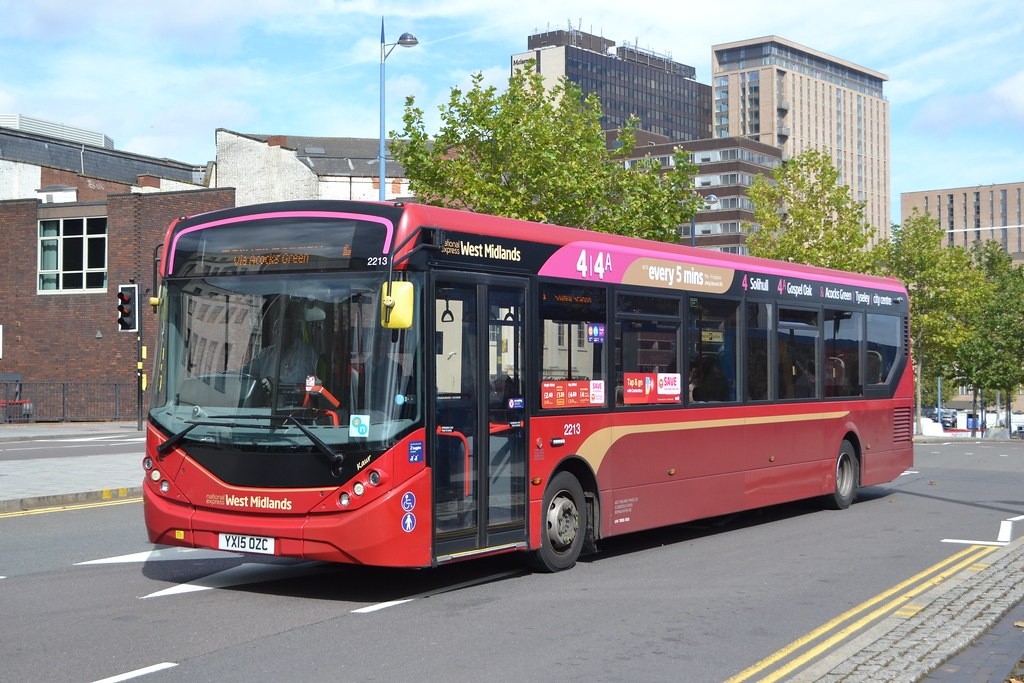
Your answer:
[935,412,957,429]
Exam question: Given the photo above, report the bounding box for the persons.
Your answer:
[687,343,836,402]
[221,316,402,407]
[492,374,522,409]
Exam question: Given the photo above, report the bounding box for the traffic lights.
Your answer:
[117,284,138,332]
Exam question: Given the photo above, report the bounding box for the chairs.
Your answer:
[866,350,883,383]
[828,356,847,396]
[295,319,329,424]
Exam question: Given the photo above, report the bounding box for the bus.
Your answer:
[142,199,914,574]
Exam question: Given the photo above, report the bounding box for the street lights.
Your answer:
[380,15,419,201]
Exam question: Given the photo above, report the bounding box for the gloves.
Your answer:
[260,376,273,396]
